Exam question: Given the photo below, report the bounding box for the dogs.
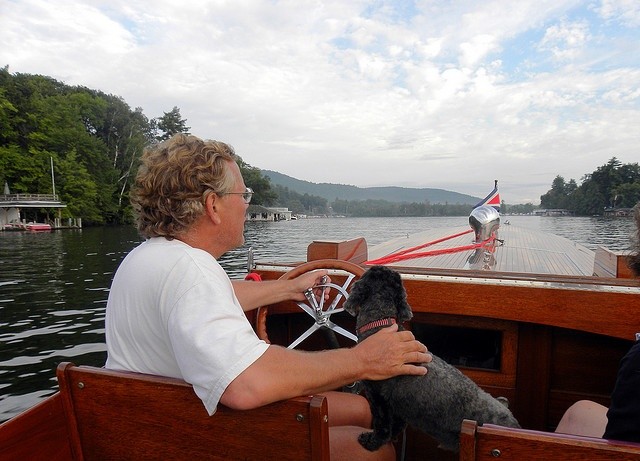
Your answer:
[342,266,522,452]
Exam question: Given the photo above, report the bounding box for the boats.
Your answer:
[5,222,51,230]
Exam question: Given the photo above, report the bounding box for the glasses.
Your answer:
[216,187,255,204]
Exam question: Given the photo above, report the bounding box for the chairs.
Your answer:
[459,417,640,461]
[56,360,330,461]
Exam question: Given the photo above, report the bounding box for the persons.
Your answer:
[551,204,640,445]
[99,130,433,461]
